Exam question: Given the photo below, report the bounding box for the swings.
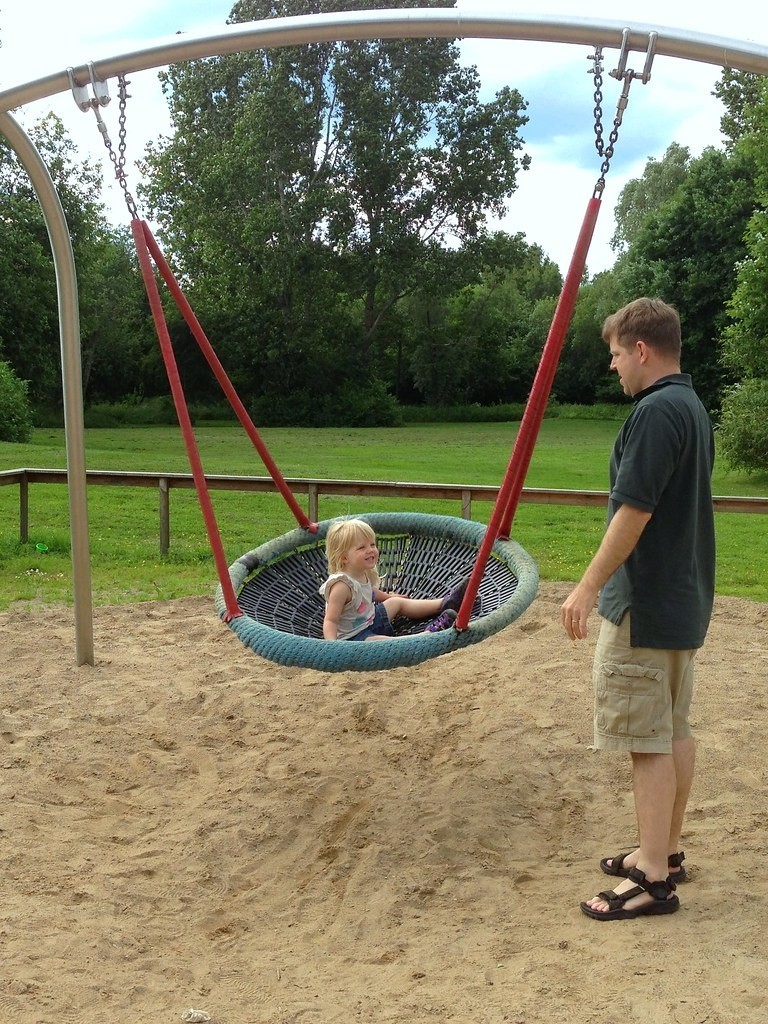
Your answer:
[88,53,631,670]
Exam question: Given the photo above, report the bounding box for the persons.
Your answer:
[558,297,720,919]
[318,517,471,643]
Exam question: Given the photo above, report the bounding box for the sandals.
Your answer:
[579,867,679,921]
[437,575,470,610]
[425,609,457,632]
[600,849,686,881]
